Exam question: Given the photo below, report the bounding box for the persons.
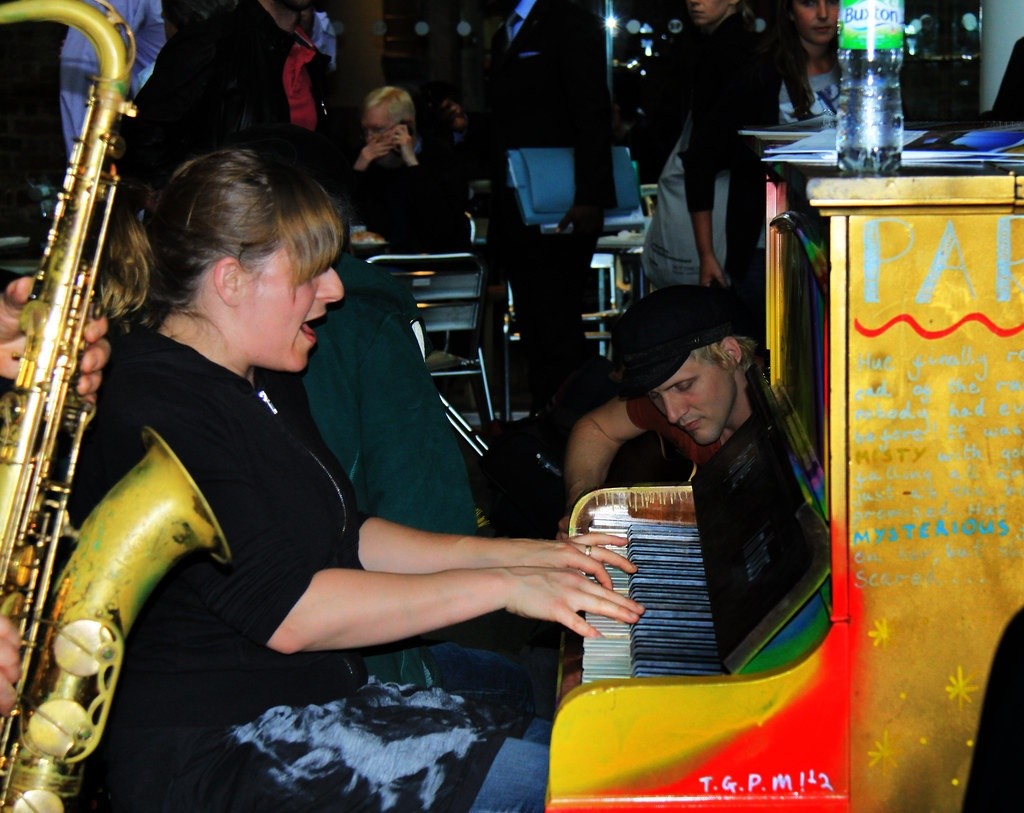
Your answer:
[55,0,1024,432]
[2,269,113,728]
[554,280,766,547]
[288,165,492,553]
[56,136,646,813]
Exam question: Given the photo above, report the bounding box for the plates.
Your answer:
[351,242,388,248]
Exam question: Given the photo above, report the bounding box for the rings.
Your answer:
[584,545,592,556]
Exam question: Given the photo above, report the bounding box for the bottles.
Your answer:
[835,0,904,172]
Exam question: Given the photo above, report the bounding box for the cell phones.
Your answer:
[394,120,415,152]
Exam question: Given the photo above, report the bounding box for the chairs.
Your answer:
[366,253,501,423]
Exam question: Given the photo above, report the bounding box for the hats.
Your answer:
[615,283,742,402]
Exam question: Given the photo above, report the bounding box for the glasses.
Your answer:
[361,123,399,139]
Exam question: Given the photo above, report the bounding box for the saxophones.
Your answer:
[0,1,236,813]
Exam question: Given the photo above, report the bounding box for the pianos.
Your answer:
[543,165,1023,813]
